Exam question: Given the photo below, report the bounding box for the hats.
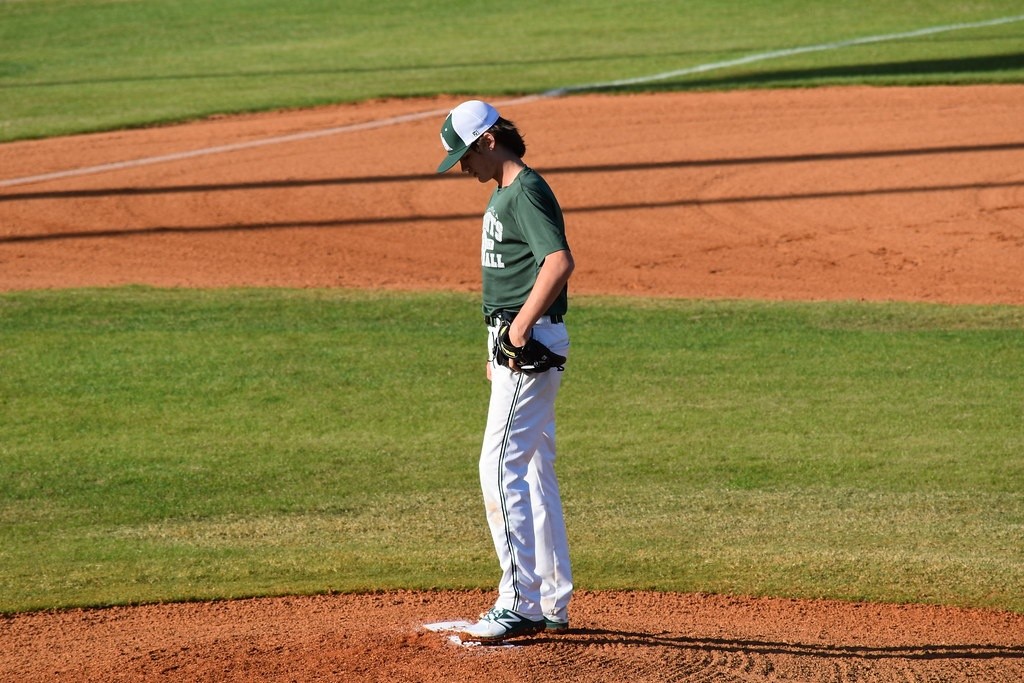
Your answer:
[436,100,500,173]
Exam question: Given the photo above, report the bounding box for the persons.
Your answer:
[438,101,577,644]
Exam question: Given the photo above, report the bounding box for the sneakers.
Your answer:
[543,615,569,634]
[460,605,547,645]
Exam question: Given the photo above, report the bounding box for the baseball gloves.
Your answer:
[493,327,569,377]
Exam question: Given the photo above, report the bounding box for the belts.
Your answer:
[485,315,563,325]
